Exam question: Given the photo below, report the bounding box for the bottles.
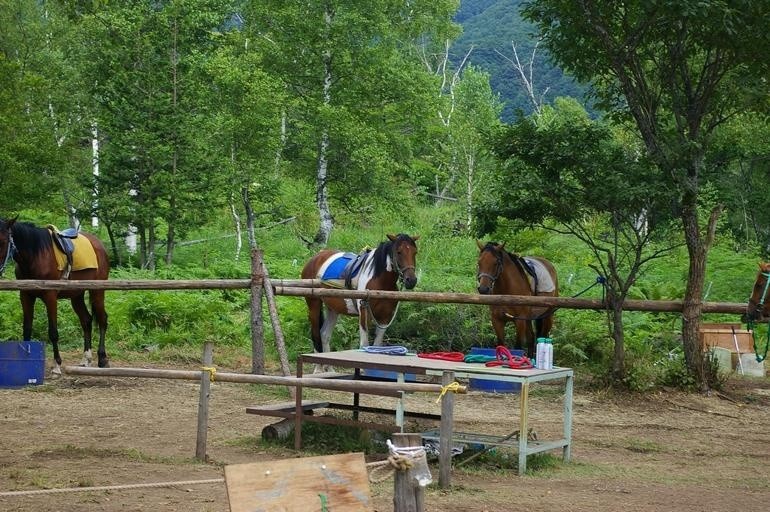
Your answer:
[545,339,554,369]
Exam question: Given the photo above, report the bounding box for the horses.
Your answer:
[0,215,110,380]
[475,238,558,360]
[746,261,770,321]
[302,233,421,374]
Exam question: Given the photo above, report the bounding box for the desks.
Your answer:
[294,349,574,475]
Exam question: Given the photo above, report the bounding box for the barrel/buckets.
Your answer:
[0,341,45,386]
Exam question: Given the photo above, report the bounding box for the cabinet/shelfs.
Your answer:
[699,323,755,370]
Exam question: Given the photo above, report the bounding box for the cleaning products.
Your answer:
[544,337,552,370]
